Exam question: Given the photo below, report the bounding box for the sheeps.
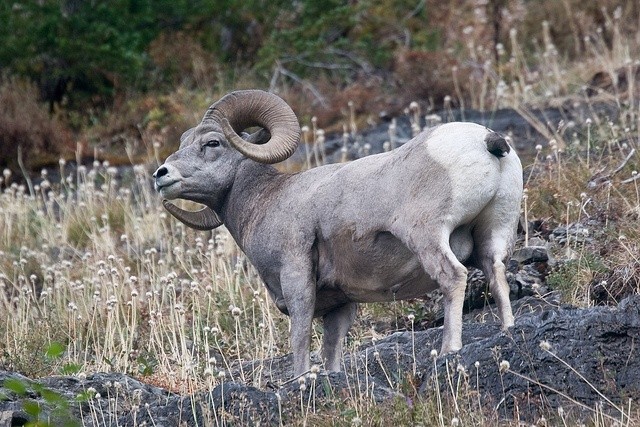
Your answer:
[152,87,524,379]
